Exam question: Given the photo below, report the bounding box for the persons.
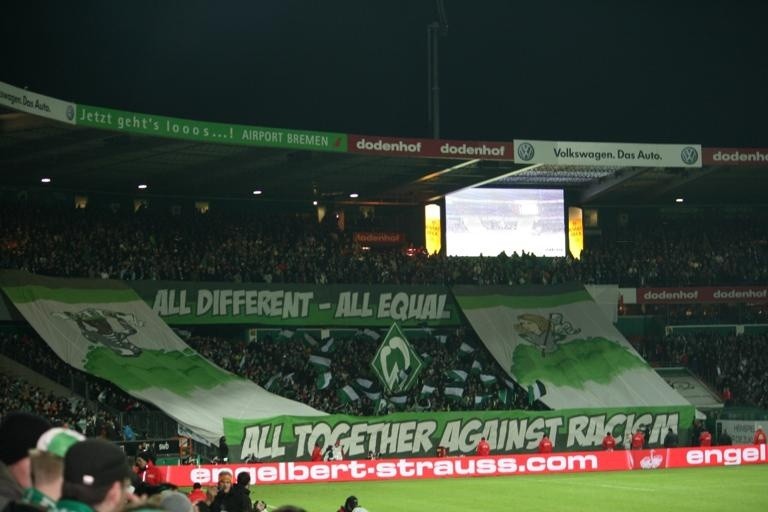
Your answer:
[538,435,552,455]
[604,432,615,451]
[183,332,545,416]
[689,420,732,446]
[1,189,768,289]
[1,322,150,440]
[664,428,680,448]
[753,425,767,444]
[0,412,365,512]
[476,438,490,456]
[631,334,768,410]
[631,429,645,450]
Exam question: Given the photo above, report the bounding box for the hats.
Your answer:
[62,439,139,487]
[352,507,367,512]
[348,496,359,510]
[36,426,87,458]
